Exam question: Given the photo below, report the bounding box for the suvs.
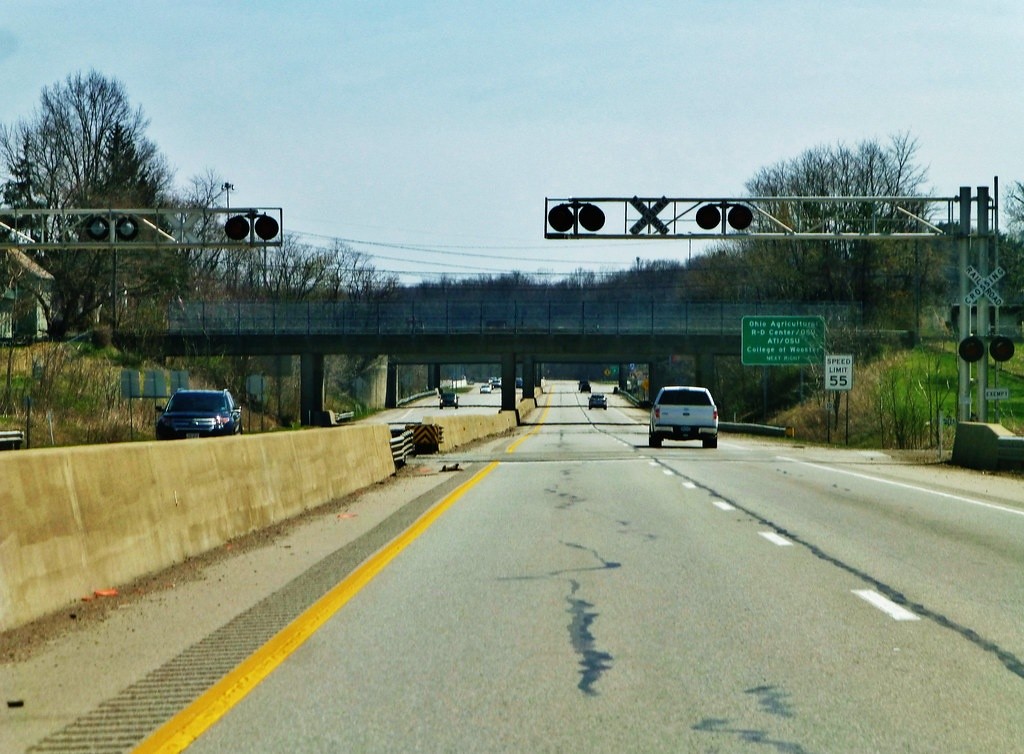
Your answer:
[154,387,243,441]
[640,385,723,448]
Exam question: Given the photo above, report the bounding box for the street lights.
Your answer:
[221,181,234,298]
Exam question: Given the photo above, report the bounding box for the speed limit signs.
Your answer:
[824,353,854,390]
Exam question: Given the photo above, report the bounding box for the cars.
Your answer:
[438,392,459,409]
[479,376,501,394]
[588,393,608,410]
[577,379,591,393]
